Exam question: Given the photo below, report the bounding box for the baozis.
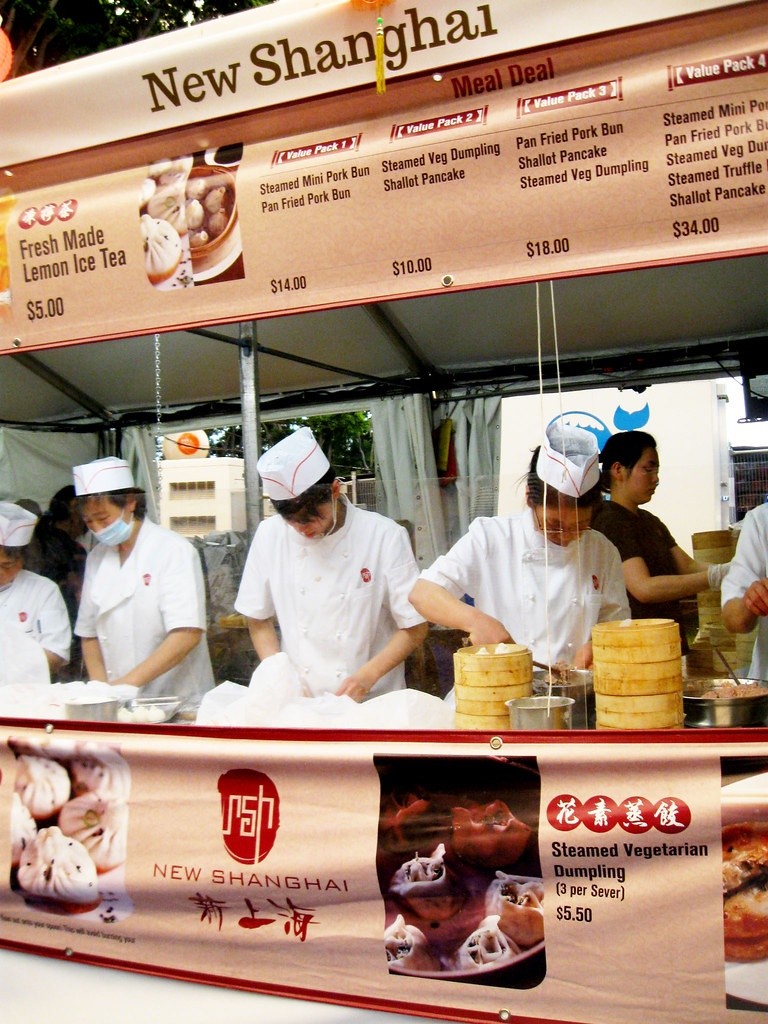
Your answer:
[140,153,226,285]
[475,642,507,655]
[10,742,127,904]
[377,791,546,971]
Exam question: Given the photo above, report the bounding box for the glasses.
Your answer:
[532,503,597,539]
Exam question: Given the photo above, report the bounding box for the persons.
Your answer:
[721,502,768,683]
[72,455,218,711]
[15,484,88,632]
[589,429,731,659]
[407,420,631,675]
[0,501,71,691]
[232,425,431,703]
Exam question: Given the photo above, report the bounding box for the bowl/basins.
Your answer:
[680,675,768,728]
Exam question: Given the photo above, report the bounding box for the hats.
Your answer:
[254,425,332,501]
[70,454,136,496]
[534,422,601,499]
[0,502,39,548]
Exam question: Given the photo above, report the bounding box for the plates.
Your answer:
[387,925,548,977]
[205,141,241,167]
[725,956,767,1005]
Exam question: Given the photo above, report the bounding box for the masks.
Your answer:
[84,508,138,548]
[76,513,89,536]
[529,525,585,568]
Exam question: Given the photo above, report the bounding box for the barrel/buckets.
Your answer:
[532,668,594,729]
[65,696,120,724]
[505,692,576,731]
